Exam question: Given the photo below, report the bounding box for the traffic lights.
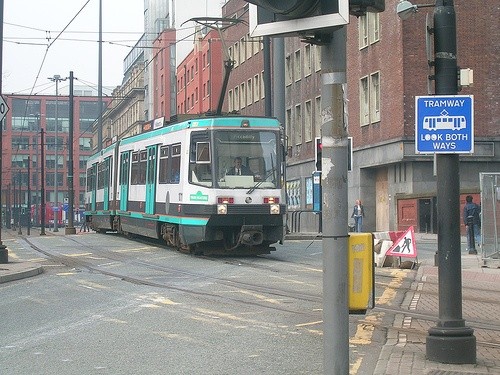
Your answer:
[315,138,323,174]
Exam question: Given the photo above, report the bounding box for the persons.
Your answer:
[226,157,261,182]
[350,200,366,232]
[82,214,91,232]
[463,195,481,251]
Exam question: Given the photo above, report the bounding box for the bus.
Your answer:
[82,111,291,259]
[31,201,63,227]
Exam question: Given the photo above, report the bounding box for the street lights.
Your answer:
[47,74,69,233]
